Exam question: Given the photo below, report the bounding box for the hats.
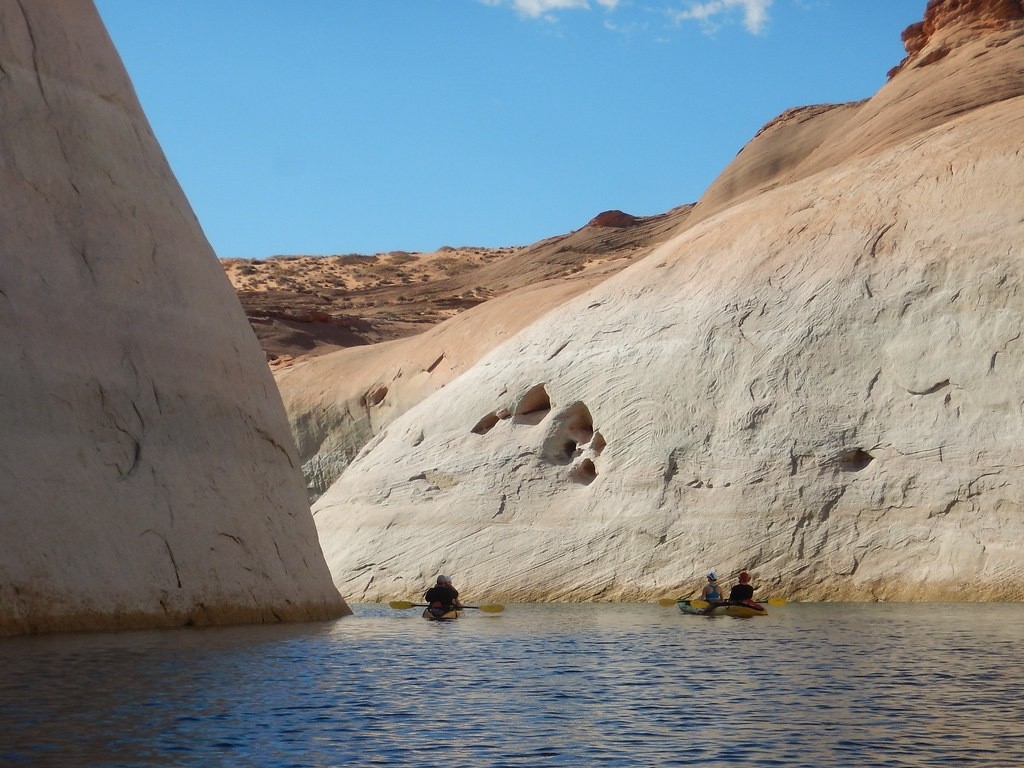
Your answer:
[739,572,750,580]
[707,572,718,579]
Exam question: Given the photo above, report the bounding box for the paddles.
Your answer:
[658,597,786,608]
[388,600,504,615]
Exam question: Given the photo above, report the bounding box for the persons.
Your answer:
[702,572,724,607]
[425,575,465,614]
[728,572,754,601]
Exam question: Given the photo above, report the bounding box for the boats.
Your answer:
[678,599,769,619]
[420,608,464,620]
